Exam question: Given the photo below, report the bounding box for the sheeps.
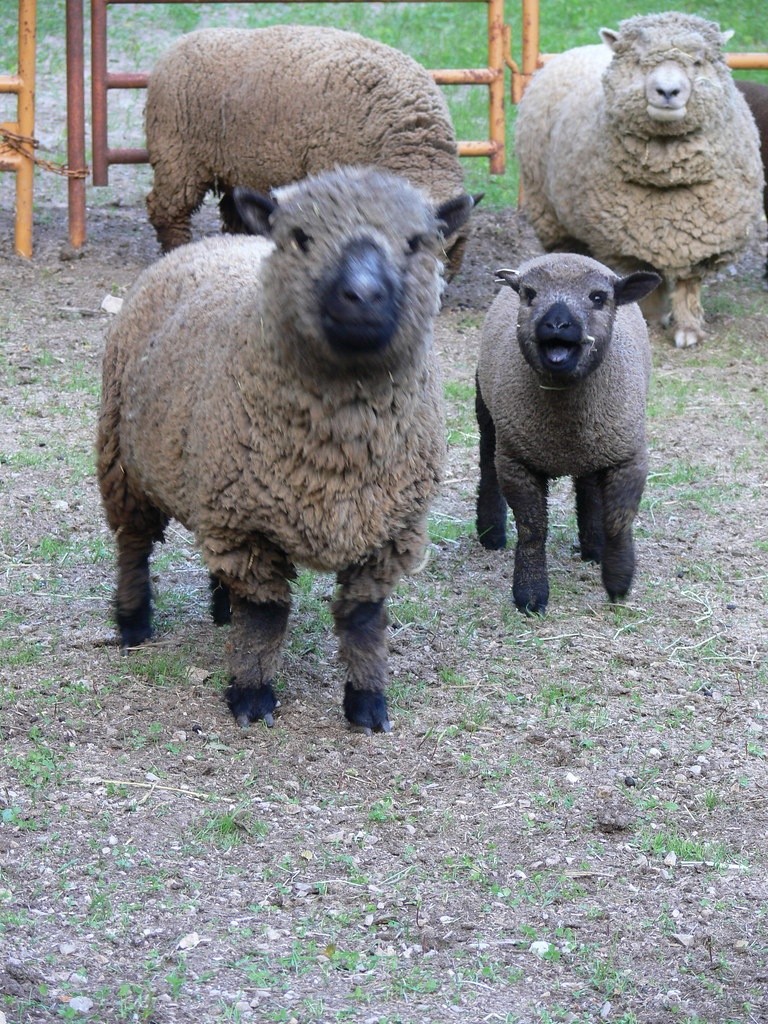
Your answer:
[515,12,768,347]
[143,25,469,284]
[97,162,485,737]
[472,252,664,618]
